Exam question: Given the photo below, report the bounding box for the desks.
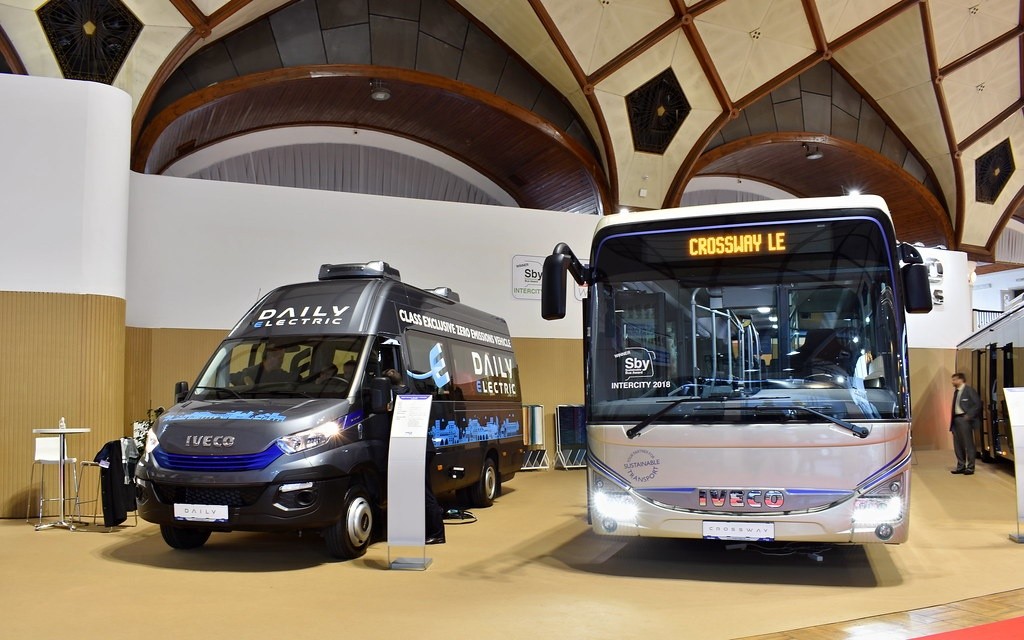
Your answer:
[33,427,91,531]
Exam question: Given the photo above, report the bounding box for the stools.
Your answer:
[71,436,138,532]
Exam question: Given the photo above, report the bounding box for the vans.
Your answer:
[132,260,524,559]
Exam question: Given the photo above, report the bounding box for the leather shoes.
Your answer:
[964,470,974,475]
[952,468,964,474]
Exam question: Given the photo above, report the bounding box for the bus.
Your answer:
[541,194,934,544]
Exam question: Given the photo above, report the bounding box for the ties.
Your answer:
[955,389,958,404]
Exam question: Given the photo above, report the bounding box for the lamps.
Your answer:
[371,79,393,102]
[802,140,825,160]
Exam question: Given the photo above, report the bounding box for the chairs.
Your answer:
[26,436,78,529]
[687,324,855,386]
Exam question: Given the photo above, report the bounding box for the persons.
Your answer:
[240,343,304,386]
[373,368,446,545]
[950,373,982,476]
[316,359,367,392]
